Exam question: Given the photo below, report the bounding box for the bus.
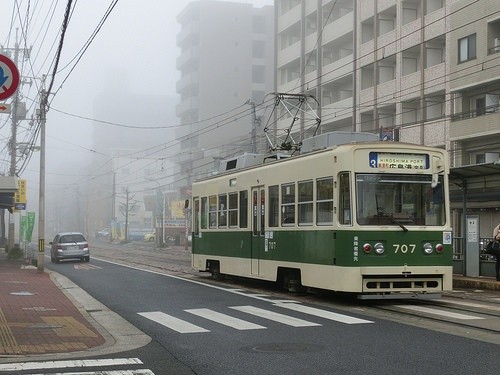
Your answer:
[191,141,453,303]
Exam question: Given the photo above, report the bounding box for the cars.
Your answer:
[49,233,91,264]
